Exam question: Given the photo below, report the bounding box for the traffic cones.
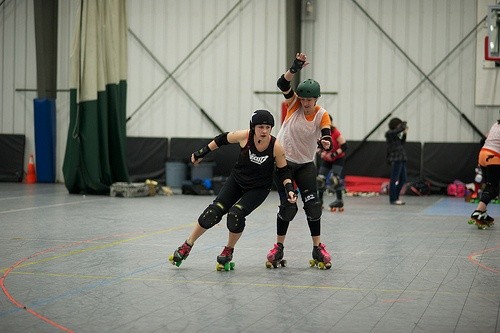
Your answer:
[25,154,37,183]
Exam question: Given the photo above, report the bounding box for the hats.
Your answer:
[389,118,402,130]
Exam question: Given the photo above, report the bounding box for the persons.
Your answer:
[317,115,345,208]
[471,120,500,223]
[267,53,335,263]
[472,164,484,198]
[169,109,298,264]
[385,117,408,205]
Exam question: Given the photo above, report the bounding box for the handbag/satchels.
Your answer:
[380,180,407,195]
[448,179,466,197]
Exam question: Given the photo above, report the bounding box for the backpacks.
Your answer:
[408,180,431,196]
[182,184,210,195]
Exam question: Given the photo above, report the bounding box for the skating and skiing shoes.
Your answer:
[169,239,193,267]
[310,242,332,270]
[469,210,494,229]
[266,242,287,268]
[217,246,235,271]
[329,201,344,212]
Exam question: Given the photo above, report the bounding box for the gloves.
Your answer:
[402,133,406,142]
[401,122,406,130]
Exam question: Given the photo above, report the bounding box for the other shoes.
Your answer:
[392,200,405,205]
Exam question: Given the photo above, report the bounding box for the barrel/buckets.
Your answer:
[165,161,186,187]
[189,162,214,188]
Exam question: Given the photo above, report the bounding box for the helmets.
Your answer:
[249,110,274,129]
[296,79,321,99]
